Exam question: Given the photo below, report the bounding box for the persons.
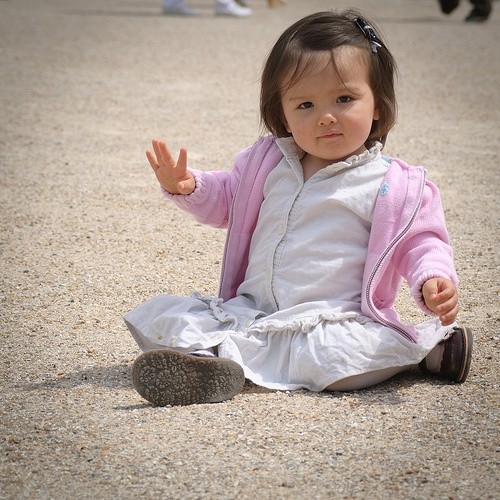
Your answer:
[123,11,474,407]
[162,0,253,17]
[438,0,493,22]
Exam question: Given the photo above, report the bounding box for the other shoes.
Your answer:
[131,348,247,406]
[436,0,460,15]
[162,0,197,16]
[464,0,493,22]
[419,327,473,384]
[213,0,254,18]
